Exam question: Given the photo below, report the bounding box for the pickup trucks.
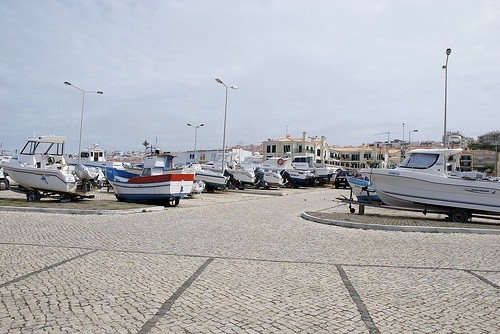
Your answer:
[335,169,362,189]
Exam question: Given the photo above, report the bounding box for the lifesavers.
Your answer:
[277,158,284,165]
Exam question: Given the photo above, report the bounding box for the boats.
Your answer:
[337,176,386,206]
[175,152,342,194]
[103,137,195,207]
[0,137,141,204]
[360,147,500,221]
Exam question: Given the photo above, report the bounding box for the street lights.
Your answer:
[64,81,103,165]
[215,77,238,174]
[442,47,452,150]
[187,123,205,164]
[408,129,418,151]
[402,123,404,144]
